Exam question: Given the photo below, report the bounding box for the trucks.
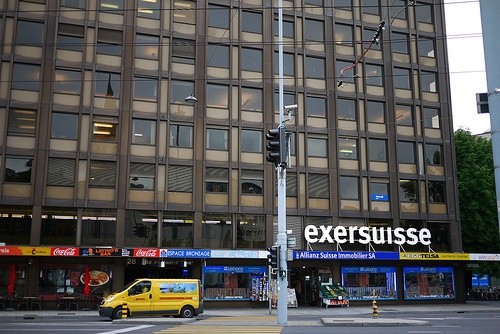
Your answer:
[97,278,204,321]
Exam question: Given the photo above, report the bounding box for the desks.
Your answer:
[63,297,74,311]
[24,296,36,310]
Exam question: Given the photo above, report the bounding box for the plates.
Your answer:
[80,271,109,285]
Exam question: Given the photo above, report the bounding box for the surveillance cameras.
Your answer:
[284,104,298,109]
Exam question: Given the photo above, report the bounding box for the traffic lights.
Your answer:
[267,126,287,164]
[265,134,279,168]
[266,248,272,267]
[269,245,280,270]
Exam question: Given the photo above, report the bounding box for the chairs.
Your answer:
[57,297,80,311]
[16,297,43,310]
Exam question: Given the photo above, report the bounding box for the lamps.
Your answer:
[185,93,198,104]
